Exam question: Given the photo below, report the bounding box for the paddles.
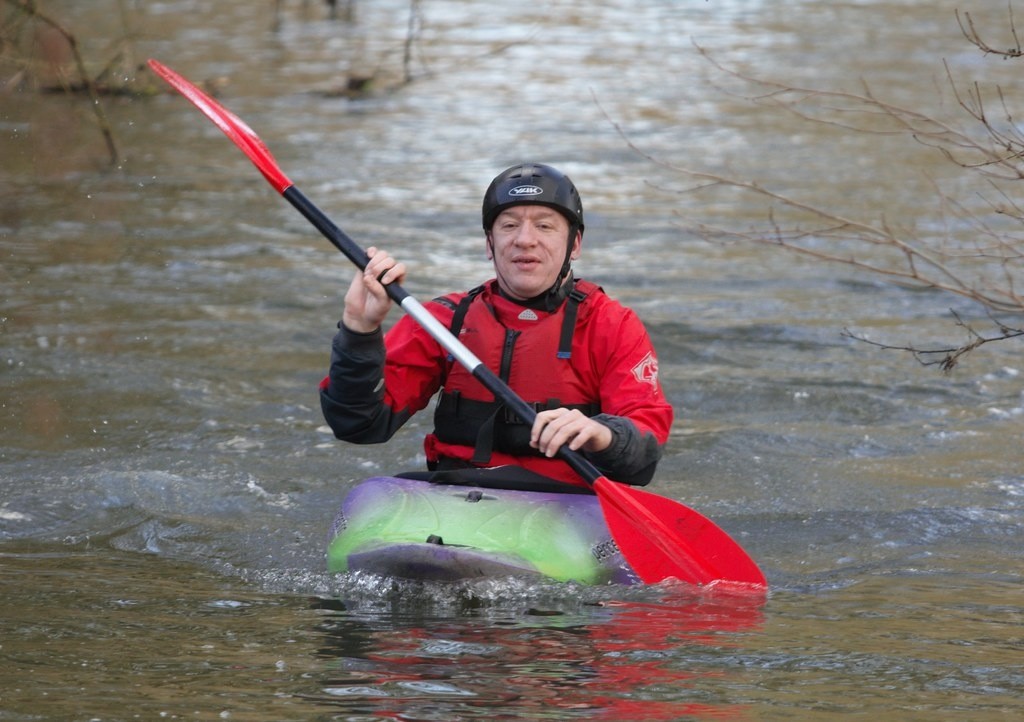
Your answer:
[146,56,768,629]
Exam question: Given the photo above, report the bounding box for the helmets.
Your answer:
[482,162,584,238]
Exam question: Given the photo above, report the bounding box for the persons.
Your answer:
[319,164,672,493]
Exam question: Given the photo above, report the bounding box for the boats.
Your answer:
[326,473,640,609]
